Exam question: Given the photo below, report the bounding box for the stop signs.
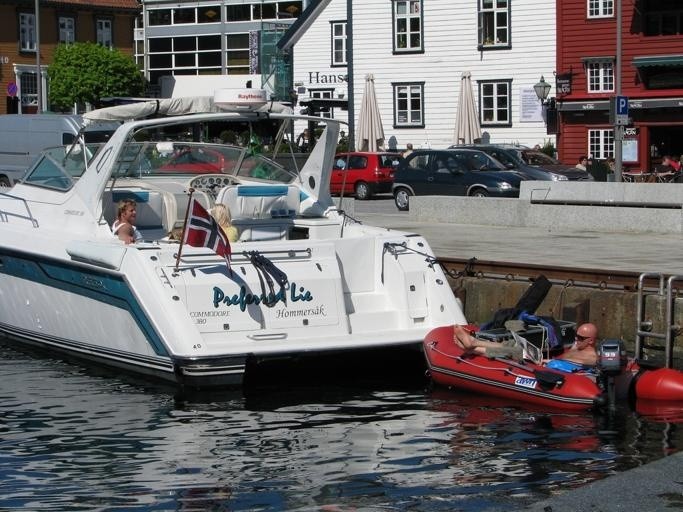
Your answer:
[5,83,19,98]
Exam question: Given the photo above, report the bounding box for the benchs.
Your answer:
[209,183,304,242]
[102,180,179,241]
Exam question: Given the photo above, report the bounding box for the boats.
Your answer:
[1,91,468,395]
[425,325,683,414]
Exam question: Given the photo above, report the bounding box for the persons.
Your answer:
[453,323,598,366]
[403,143,417,168]
[576,156,588,171]
[654,155,676,184]
[112,199,145,244]
[212,204,238,242]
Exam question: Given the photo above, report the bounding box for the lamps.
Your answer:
[533,75,551,107]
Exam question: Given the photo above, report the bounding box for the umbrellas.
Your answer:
[355,73,384,151]
[455,71,482,144]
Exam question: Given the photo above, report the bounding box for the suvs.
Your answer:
[449,143,593,181]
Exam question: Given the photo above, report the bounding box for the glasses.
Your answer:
[574,333,592,341]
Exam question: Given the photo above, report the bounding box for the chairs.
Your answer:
[436,157,460,175]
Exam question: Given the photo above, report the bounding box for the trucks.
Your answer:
[1,112,152,189]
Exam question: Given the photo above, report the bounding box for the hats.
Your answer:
[505,320,526,333]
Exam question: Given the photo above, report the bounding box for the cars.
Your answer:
[390,149,531,212]
[330,151,411,201]
